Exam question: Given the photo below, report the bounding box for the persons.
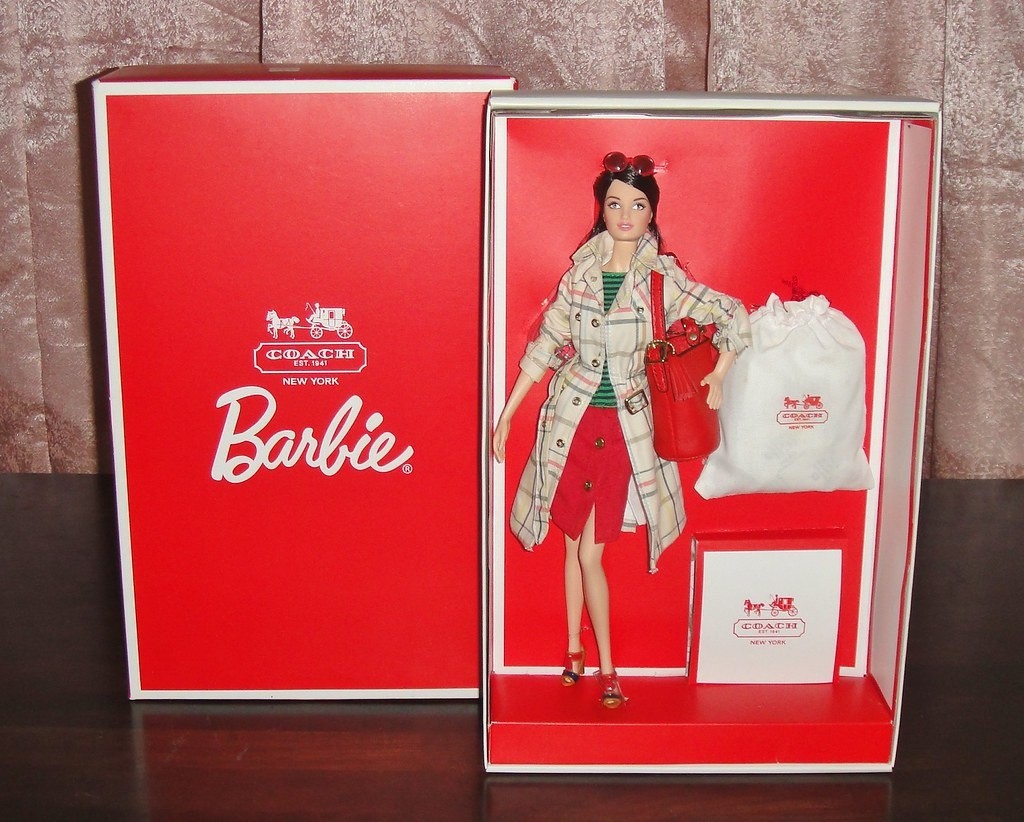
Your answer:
[491,153,754,713]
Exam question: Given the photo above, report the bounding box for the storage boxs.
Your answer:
[481,92,943,774]
[92,62,517,704]
